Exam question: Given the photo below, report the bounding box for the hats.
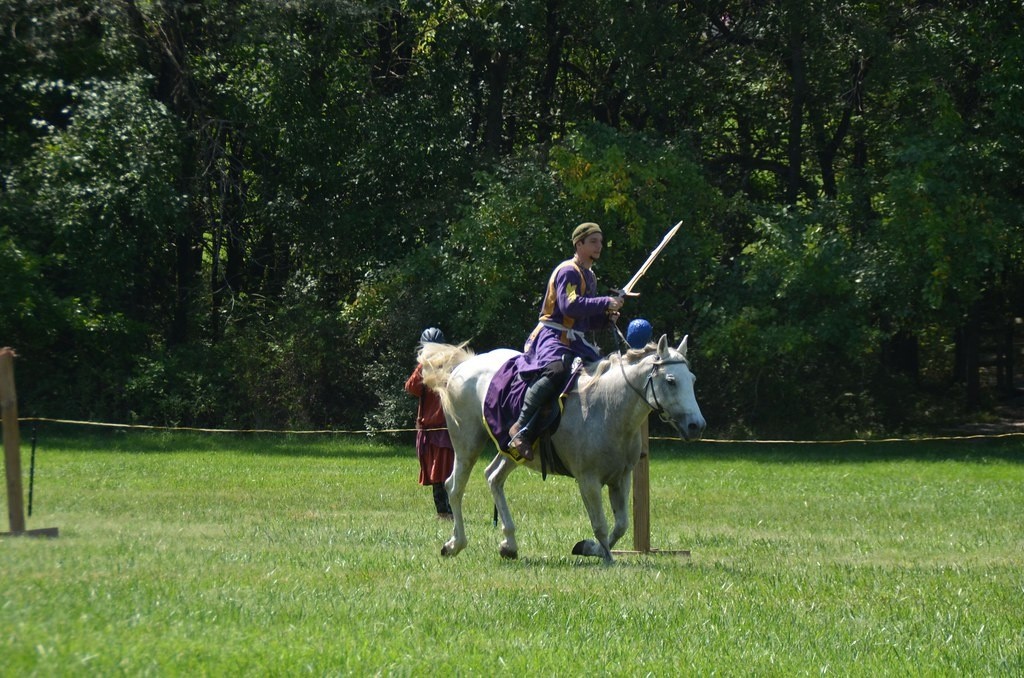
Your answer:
[571,222,602,247]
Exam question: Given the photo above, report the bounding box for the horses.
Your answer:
[418,330,706,568]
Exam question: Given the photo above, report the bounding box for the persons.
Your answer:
[406,327,456,515]
[510,221,623,460]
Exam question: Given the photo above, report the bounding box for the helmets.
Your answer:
[420,328,446,343]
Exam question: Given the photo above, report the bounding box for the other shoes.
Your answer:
[509,423,535,462]
